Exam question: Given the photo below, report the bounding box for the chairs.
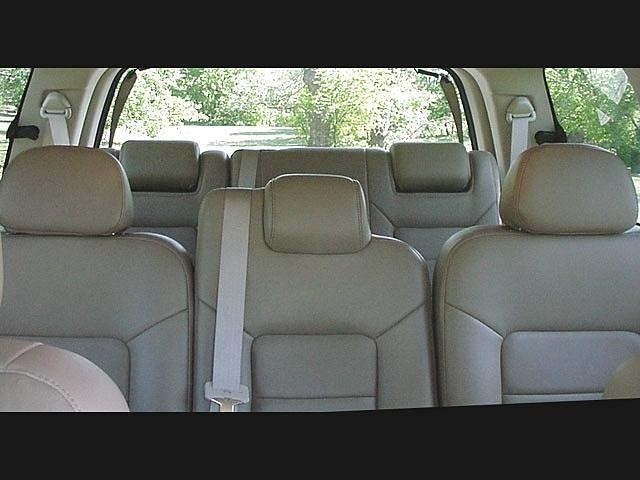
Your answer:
[0,139,640,412]
